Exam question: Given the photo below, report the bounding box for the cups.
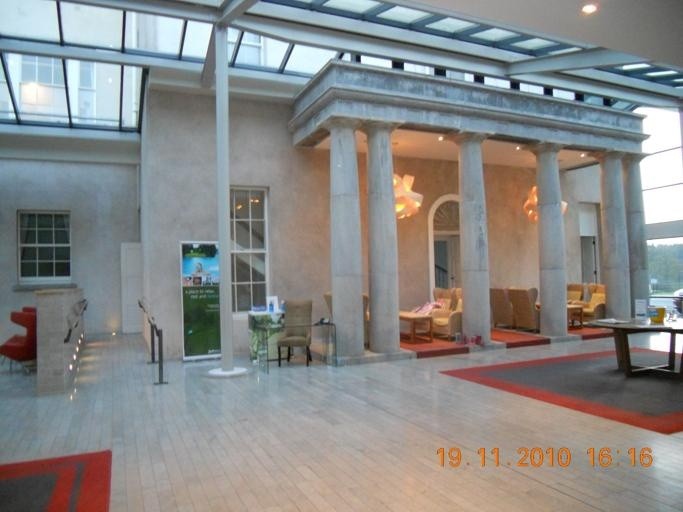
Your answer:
[648,306,667,325]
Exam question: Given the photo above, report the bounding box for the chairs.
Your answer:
[276,298,313,367]
[489,281,606,333]
[323,291,370,348]
[414,287,463,343]
[0,306,36,378]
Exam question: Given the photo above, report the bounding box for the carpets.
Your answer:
[399,332,469,358]
[0,449,112,512]
[438,346,683,435]
[491,327,550,348]
[566,323,615,339]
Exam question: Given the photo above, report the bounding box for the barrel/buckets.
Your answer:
[648,305,665,323]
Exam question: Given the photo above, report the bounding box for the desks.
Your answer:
[583,316,683,381]
[248,315,338,376]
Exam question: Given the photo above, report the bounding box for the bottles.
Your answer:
[268,300,286,312]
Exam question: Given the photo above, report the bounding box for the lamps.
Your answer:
[392,172,423,218]
[523,186,567,222]
